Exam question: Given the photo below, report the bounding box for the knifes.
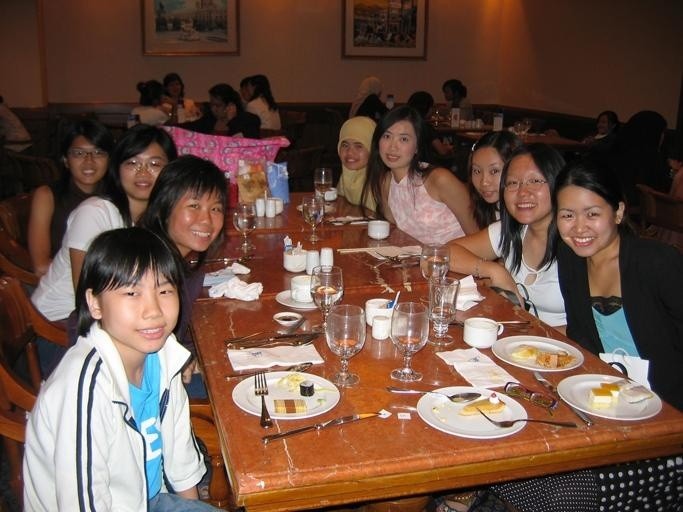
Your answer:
[226,331,322,345]
[532,372,592,427]
[447,320,531,326]
[189,255,263,263]
[261,411,382,444]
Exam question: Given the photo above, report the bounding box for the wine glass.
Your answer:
[513,118,531,141]
[427,275,459,345]
[418,243,451,303]
[314,169,332,213]
[233,202,258,251]
[326,306,367,384]
[310,266,343,332]
[389,303,429,383]
[301,194,324,243]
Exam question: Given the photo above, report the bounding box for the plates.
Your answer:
[492,334,585,373]
[557,374,662,421]
[416,384,527,439]
[297,202,334,215]
[276,291,341,310]
[231,369,342,422]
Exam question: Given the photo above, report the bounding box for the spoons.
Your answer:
[389,388,480,402]
[238,333,318,350]
[222,360,311,380]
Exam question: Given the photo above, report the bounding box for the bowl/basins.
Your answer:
[272,313,302,328]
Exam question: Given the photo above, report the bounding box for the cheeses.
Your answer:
[275,400,307,413]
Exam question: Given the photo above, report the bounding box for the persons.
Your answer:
[138,154,227,386]
[335,76,683,245]
[32,122,178,374]
[436,155,682,511]
[124,74,281,141]
[433,141,569,336]
[24,227,219,512]
[27,117,126,278]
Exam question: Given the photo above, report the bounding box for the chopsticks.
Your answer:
[337,247,378,253]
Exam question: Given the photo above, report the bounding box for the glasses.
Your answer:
[505,176,548,194]
[66,148,105,158]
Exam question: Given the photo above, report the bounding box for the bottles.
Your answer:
[370,314,390,340]
[431,105,481,129]
[171,96,185,123]
[305,250,320,275]
[320,247,333,272]
[255,197,283,217]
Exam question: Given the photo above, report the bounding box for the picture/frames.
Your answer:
[340,0,428,61]
[140,0,240,56]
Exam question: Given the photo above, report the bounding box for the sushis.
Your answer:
[300,381,314,395]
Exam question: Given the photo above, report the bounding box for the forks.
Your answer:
[252,371,271,429]
[225,312,307,343]
[476,408,577,428]
[375,252,424,262]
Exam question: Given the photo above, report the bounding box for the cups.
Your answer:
[315,187,338,203]
[462,317,502,349]
[366,219,390,242]
[289,276,320,302]
[365,299,399,326]
[283,250,306,274]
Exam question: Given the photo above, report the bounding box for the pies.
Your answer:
[465,393,505,415]
[536,353,572,368]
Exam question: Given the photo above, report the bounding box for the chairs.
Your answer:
[631,184,683,219]
[1,190,229,511]
